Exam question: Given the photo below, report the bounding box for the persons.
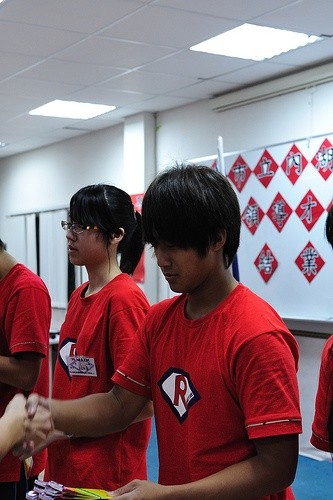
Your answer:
[0,232,49,500]
[18,165,302,500]
[43,184,152,493]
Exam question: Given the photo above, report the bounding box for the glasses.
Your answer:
[61,221,100,233]
